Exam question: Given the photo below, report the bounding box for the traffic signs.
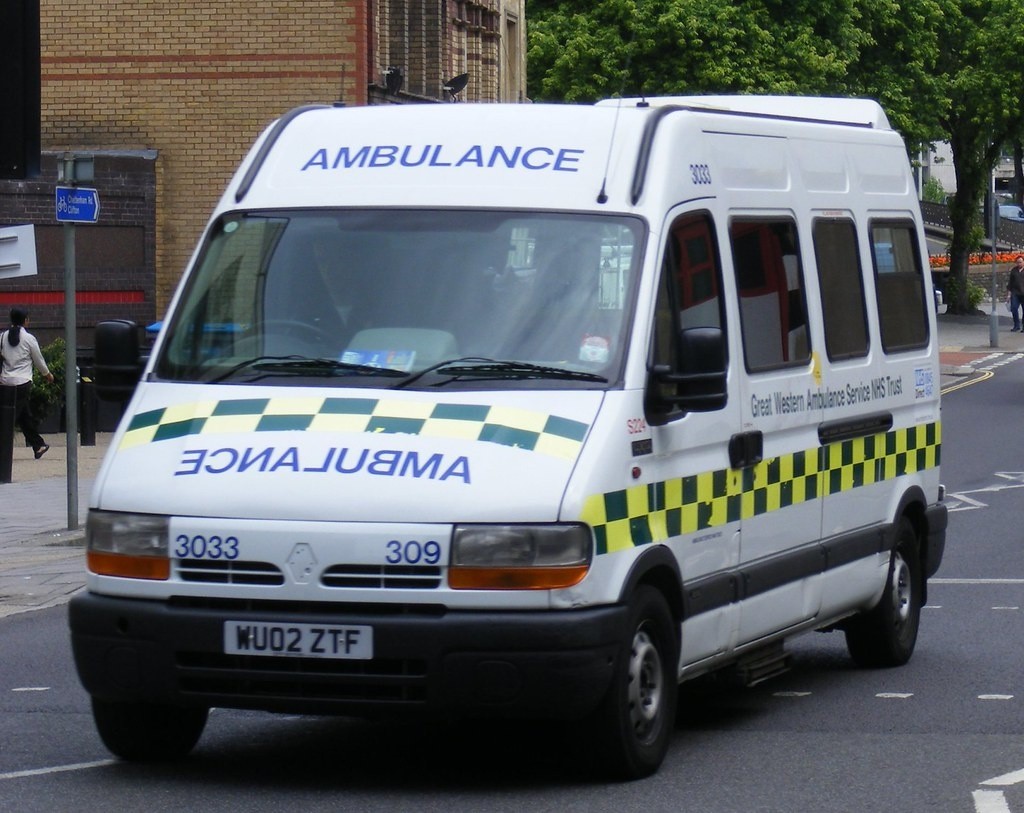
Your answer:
[54,187,101,225]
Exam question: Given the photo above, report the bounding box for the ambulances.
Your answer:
[69,99,945,785]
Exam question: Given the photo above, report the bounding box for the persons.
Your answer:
[1005,256,1024,332]
[0,304,55,459]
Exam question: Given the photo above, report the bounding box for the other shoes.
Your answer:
[1021,328,1024,332]
[35,445,49,459]
[1011,327,1020,331]
